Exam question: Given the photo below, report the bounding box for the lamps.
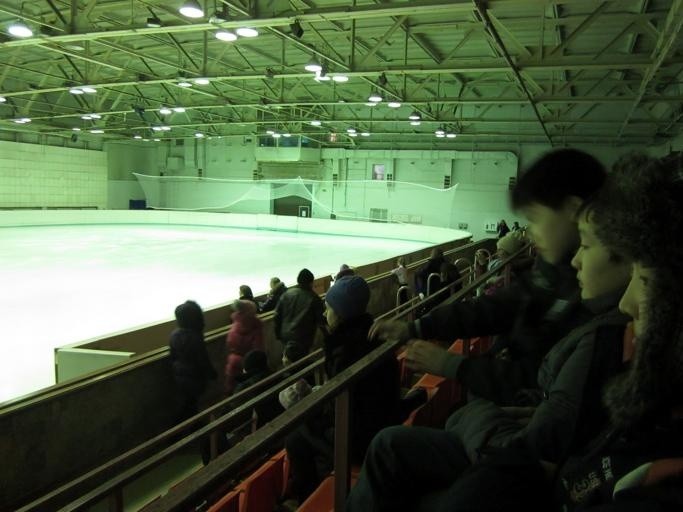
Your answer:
[266,128,292,140]
[13,118,32,127]
[346,127,371,139]
[211,24,262,45]
[176,77,212,90]
[3,17,34,42]
[409,110,457,140]
[314,69,348,84]
[194,132,207,140]
[367,91,382,104]
[156,106,185,117]
[67,86,96,97]
[302,55,325,73]
[80,113,100,123]
[177,1,206,20]
[387,99,401,109]
[152,124,173,134]
[310,119,322,128]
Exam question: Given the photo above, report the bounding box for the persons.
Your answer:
[161,143,683,512]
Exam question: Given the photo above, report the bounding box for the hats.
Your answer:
[271,277,284,295]
[324,275,370,321]
[278,378,311,410]
[496,231,525,254]
[293,263,313,284]
[234,299,258,314]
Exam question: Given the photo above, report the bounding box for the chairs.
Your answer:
[137,337,490,512]
